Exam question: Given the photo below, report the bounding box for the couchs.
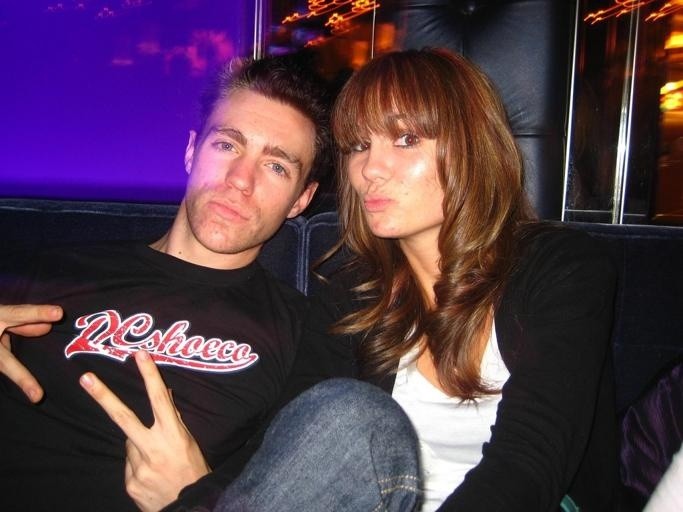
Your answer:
[0,196,683,405]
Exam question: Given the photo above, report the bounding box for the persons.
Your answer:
[645,444,683,512]
[620,350,683,512]
[0,57,356,511]
[156,48,621,510]
